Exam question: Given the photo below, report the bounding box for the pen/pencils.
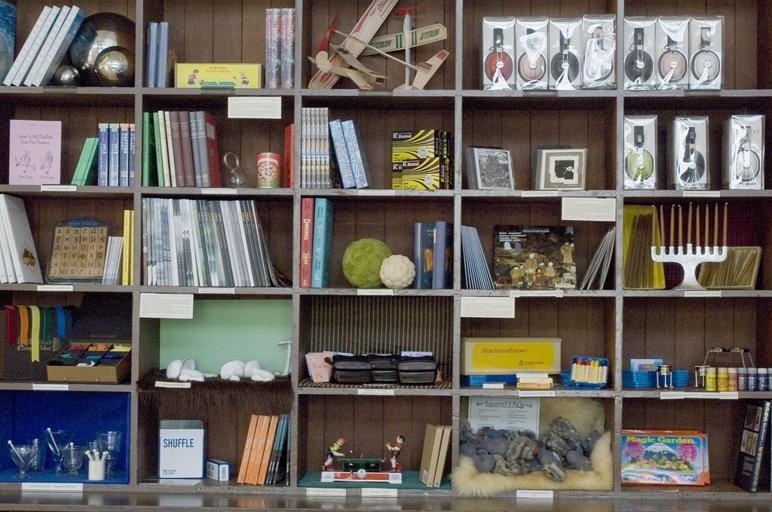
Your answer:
[571,356,608,385]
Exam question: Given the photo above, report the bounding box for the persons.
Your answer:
[320,438,347,470]
[386,435,404,472]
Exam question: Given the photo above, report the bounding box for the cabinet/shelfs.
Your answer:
[1,0,772,500]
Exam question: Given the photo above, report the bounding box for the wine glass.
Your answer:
[7,429,127,481]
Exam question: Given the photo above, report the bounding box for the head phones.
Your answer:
[731,125,761,182]
[550,27,579,84]
[624,27,654,83]
[691,27,720,82]
[624,125,654,182]
[585,26,614,82]
[658,28,688,83]
[485,28,513,84]
[518,28,546,84]
[675,126,705,184]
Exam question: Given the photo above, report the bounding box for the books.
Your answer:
[391,129,453,190]
[148,21,180,87]
[265,9,295,88]
[302,107,372,189]
[420,424,451,488]
[301,197,333,288]
[413,221,452,290]
[3,6,135,287]
[142,197,289,288]
[237,414,291,485]
[142,110,222,188]
[730,399,772,494]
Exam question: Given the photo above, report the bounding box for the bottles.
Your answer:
[704,366,771,392]
[256,152,280,188]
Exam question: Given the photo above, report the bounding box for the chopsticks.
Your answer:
[701,251,757,286]
[625,213,653,288]
